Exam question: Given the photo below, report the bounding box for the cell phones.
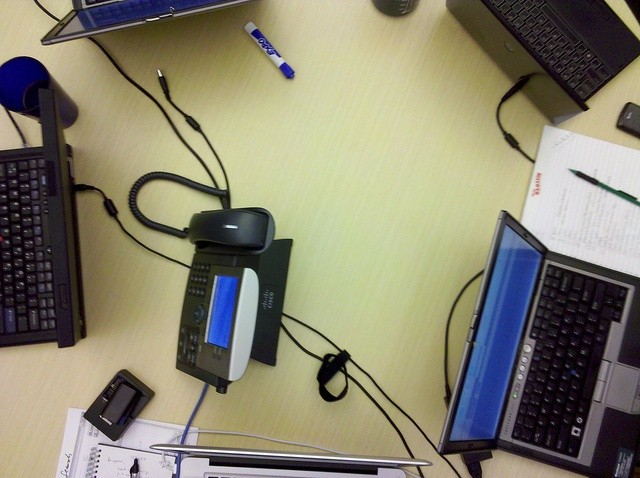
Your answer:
[615,101,640,137]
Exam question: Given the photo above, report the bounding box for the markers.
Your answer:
[245,22,296,81]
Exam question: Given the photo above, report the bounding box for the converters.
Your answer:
[443,393,494,477]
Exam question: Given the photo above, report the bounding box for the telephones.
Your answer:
[129,171,293,395]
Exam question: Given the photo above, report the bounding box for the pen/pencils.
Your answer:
[568,169,640,206]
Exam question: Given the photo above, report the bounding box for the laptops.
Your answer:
[149,444,432,477]
[40,0,249,50]
[437,209,640,477]
[0,87,88,349]
[445,0,640,126]
